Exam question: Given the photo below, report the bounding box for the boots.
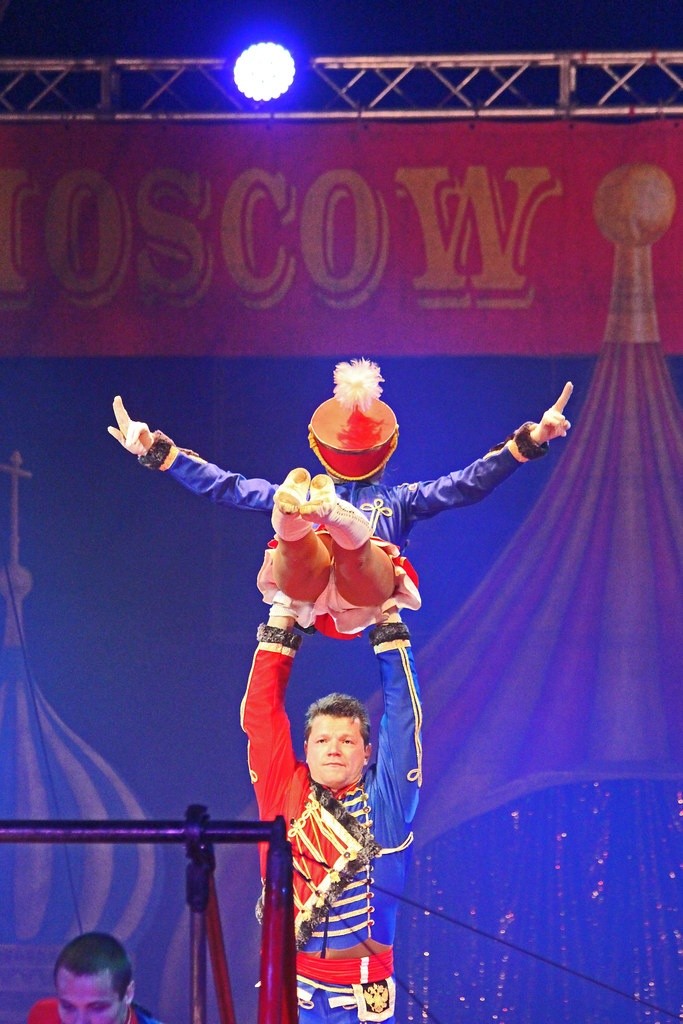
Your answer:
[271,466,311,541]
[299,475,373,549]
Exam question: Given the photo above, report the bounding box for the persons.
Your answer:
[26,931,165,1024]
[238,547,428,1024]
[105,379,575,644]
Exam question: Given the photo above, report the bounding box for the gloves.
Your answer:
[108,395,154,456]
[269,604,297,619]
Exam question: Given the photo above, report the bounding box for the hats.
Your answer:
[309,357,400,480]
